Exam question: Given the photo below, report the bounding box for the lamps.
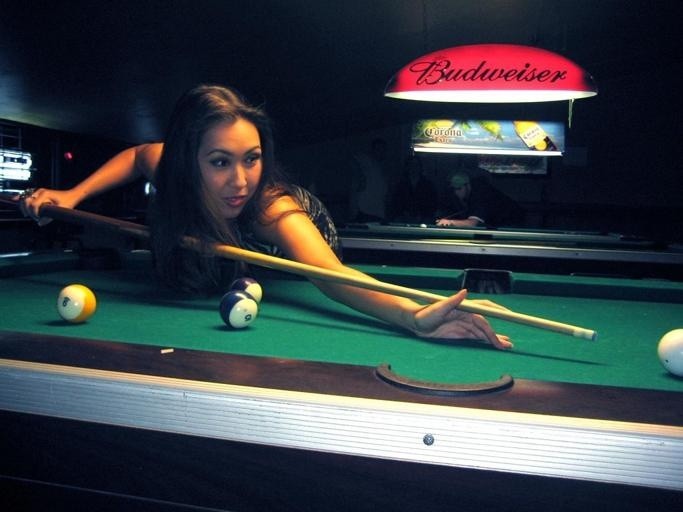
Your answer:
[407,118,567,161]
[378,1,599,105]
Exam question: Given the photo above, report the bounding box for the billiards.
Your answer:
[406,224,410,227]
[57,284,96,323]
[219,277,266,330]
[658,329,683,377]
[420,223,427,228]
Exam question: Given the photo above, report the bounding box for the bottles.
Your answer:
[512,120,558,151]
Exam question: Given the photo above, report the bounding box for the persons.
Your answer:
[436,172,485,227]
[12,85,513,349]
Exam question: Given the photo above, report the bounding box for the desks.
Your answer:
[332,217,670,253]
[0,253,682,511]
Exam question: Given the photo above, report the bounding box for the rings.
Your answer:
[31,192,38,199]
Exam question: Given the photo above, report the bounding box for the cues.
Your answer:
[435,209,465,224]
[1,192,596,342]
